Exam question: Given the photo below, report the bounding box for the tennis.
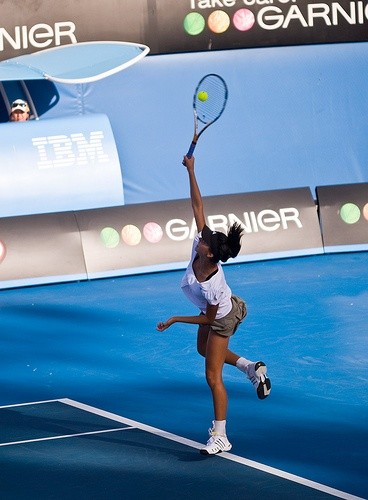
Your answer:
[197,91,208,103]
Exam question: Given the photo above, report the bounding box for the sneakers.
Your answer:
[199,427,231,457]
[246,361,271,399]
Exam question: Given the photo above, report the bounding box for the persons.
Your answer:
[11,99,29,122]
[155,154,271,455]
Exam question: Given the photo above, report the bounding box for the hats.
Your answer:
[10,99,30,113]
[201,224,229,262]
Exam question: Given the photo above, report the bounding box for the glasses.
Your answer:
[10,103,26,107]
